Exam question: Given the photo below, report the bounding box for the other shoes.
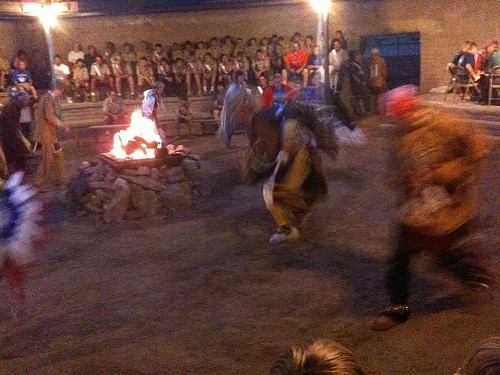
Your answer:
[204,91,214,96]
[370,304,410,331]
[294,197,314,228]
[187,93,192,97]
[52,180,63,192]
[66,91,143,104]
[35,184,50,193]
[197,92,202,97]
[269,224,300,244]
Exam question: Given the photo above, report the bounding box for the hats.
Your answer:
[383,82,418,117]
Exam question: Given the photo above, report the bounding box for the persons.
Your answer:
[32,79,70,192]
[261,103,331,247]
[0,48,40,116]
[0,91,35,178]
[103,89,129,136]
[49,30,351,126]
[173,92,195,142]
[219,69,258,150]
[269,336,366,375]
[454,334,500,375]
[141,80,167,146]
[347,49,369,117]
[367,48,393,111]
[372,83,497,328]
[446,40,499,104]
[0,141,11,187]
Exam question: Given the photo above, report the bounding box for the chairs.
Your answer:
[488,65,500,106]
[444,65,483,105]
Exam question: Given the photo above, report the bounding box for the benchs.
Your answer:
[0,89,220,144]
